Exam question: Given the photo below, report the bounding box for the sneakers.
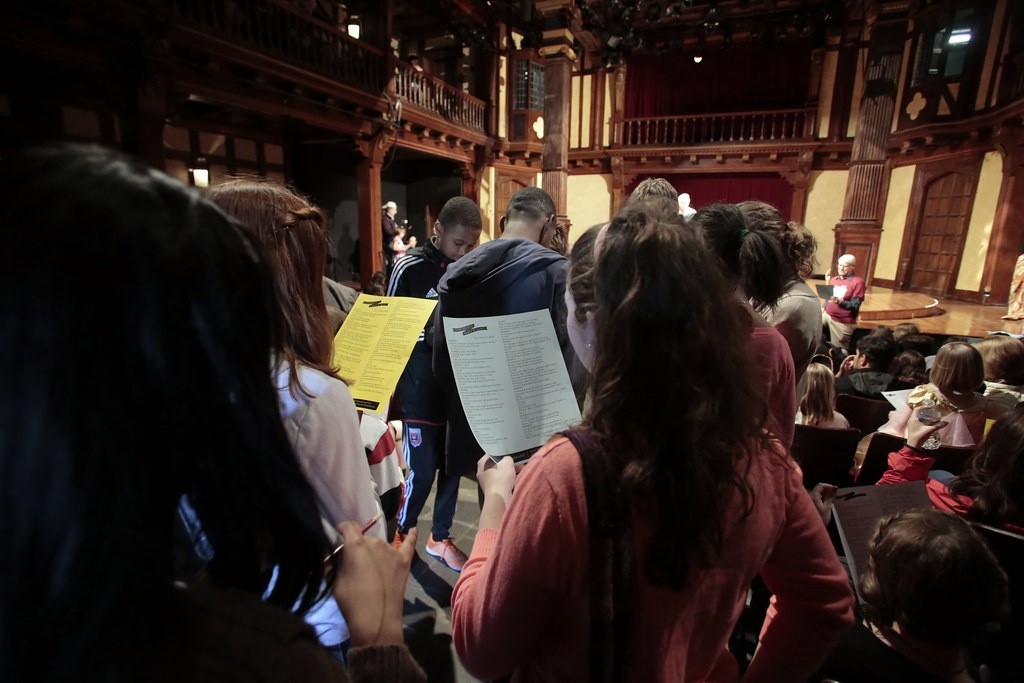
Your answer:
[394,530,406,551]
[425,532,468,571]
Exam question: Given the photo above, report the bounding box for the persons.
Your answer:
[681,204,797,455]
[451,199,855,651]
[383,195,470,572]
[0,142,431,683]
[631,177,677,202]
[171,178,408,647]
[808,483,1024,683]
[822,254,866,355]
[795,322,1024,536]
[246,0,317,63]
[432,186,591,511]
[739,201,822,406]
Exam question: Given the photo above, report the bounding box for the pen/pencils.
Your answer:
[322,514,381,563]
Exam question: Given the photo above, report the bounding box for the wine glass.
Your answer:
[917,397,941,451]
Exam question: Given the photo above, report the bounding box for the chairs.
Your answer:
[730,324,984,679]
